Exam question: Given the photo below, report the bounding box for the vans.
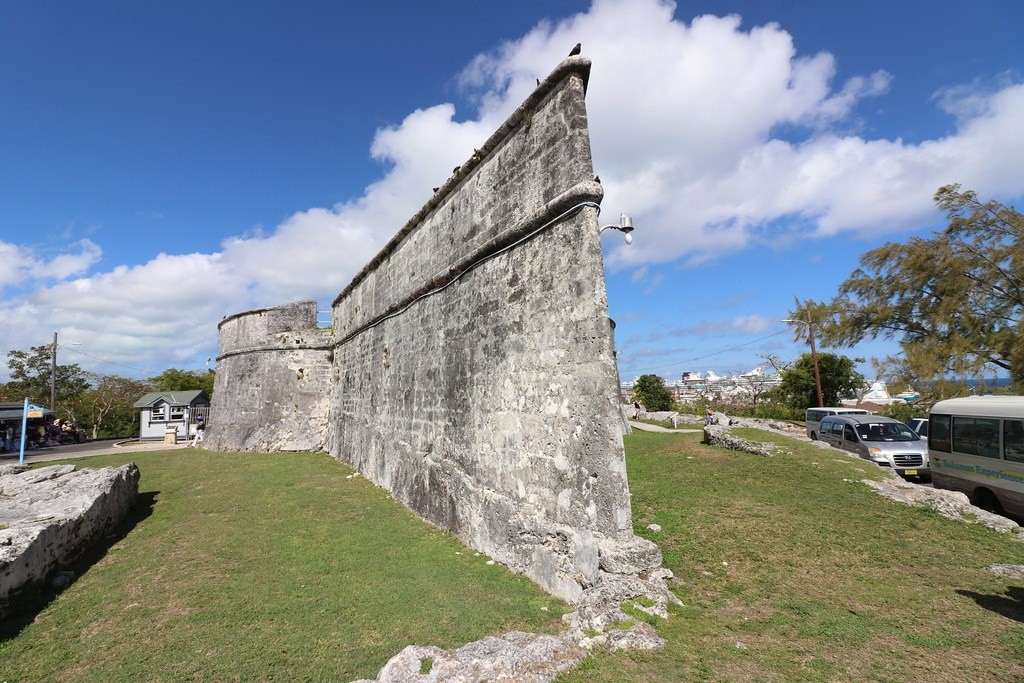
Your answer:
[819,415,932,482]
[806,407,871,443]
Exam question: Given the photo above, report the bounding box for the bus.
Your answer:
[928,394,1024,522]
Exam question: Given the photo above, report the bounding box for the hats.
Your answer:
[197,415,203,418]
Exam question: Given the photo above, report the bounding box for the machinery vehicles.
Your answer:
[44,422,86,445]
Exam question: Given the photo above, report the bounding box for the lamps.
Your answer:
[600,213,635,245]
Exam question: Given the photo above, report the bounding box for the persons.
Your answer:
[634,400,640,420]
[5,423,16,452]
[191,416,205,446]
[705,407,713,426]
